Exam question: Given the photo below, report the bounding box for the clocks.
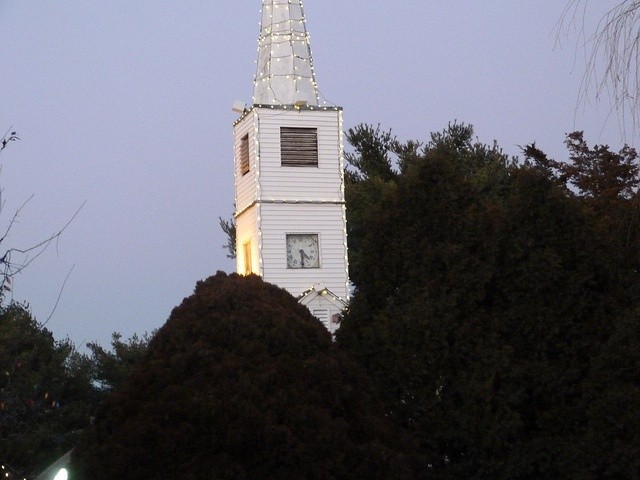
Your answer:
[285,233,319,269]
